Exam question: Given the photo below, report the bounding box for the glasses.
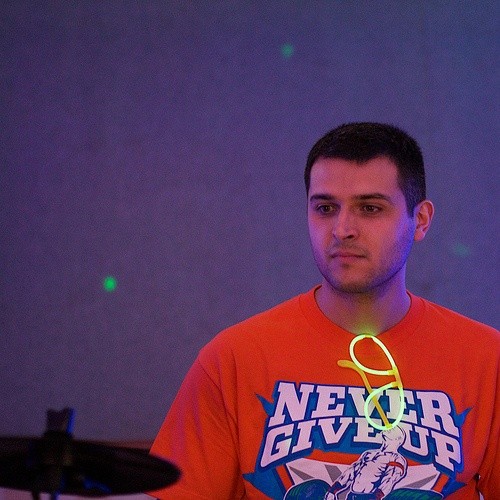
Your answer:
[336,333,405,431]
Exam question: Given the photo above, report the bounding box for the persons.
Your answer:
[136,121,499,500]
